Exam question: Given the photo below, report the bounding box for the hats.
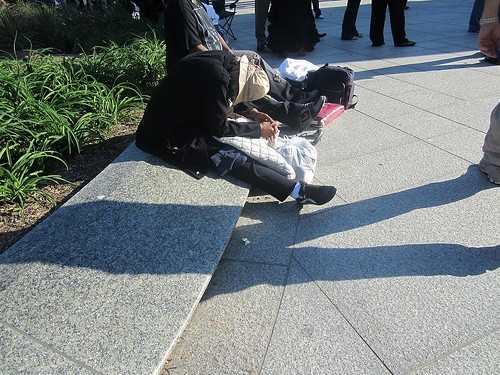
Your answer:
[232,55,270,106]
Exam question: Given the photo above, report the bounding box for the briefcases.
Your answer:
[306,64,357,109]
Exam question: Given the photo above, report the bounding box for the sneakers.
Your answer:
[296,181,337,205]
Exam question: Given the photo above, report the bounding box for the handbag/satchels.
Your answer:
[274,134,318,184]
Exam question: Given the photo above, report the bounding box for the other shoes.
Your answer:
[318,32,327,38]
[371,42,386,46]
[298,95,326,131]
[398,40,416,46]
[350,34,363,40]
[318,14,325,19]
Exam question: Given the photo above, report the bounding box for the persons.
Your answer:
[134,49,337,206]
[254,0,270,52]
[341,0,362,40]
[165,0,326,133]
[477,0,500,65]
[305,0,327,38]
[478,101,500,185]
[466,0,486,32]
[312,0,324,19]
[370,0,416,47]
[404,0,409,10]
